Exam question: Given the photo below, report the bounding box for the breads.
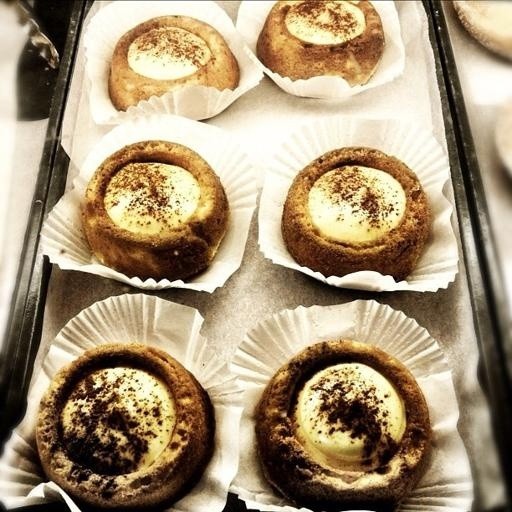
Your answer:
[256,0,385,87]
[491,95,511,179]
[451,0,512,64]
[254,339,431,512]
[281,147,433,283]
[81,140,229,280]
[35,342,214,511]
[109,16,241,117]
